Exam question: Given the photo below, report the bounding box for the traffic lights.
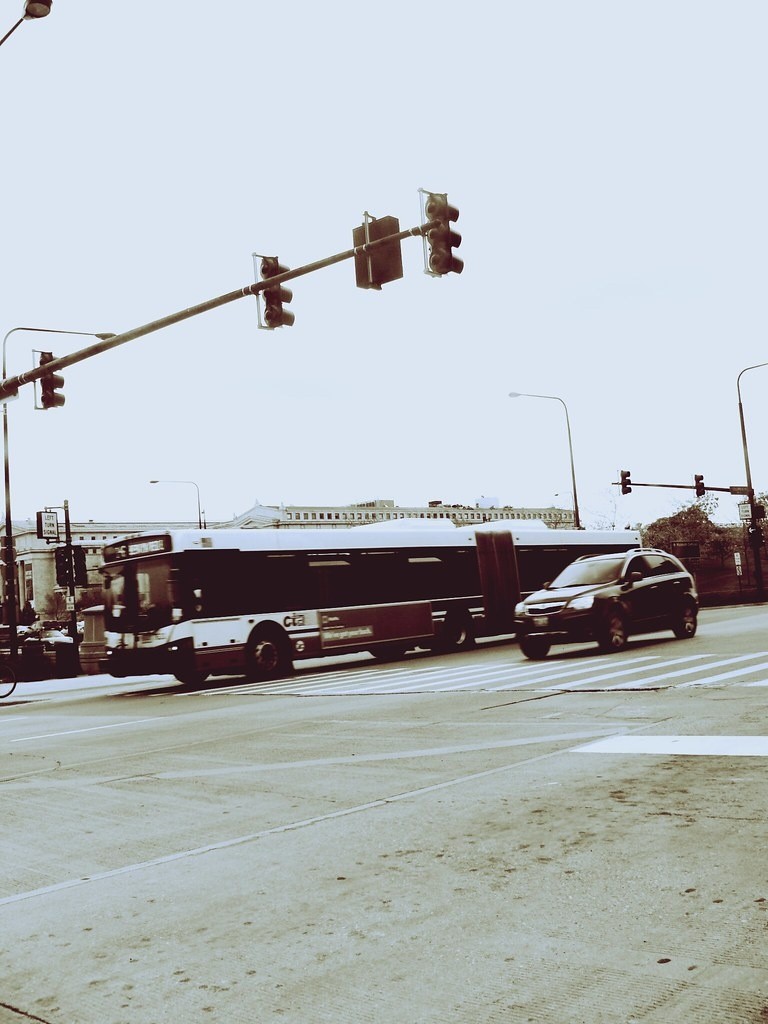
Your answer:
[425,193,464,276]
[260,255,294,329]
[695,475,705,497]
[38,352,66,410]
[621,470,633,495]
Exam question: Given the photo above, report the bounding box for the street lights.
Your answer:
[508,392,580,530]
[150,480,201,530]
[0,324,116,682]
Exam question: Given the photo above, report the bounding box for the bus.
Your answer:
[99,517,645,688]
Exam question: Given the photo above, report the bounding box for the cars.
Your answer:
[0,620,86,652]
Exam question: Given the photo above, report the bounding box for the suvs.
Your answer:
[511,547,700,660]
